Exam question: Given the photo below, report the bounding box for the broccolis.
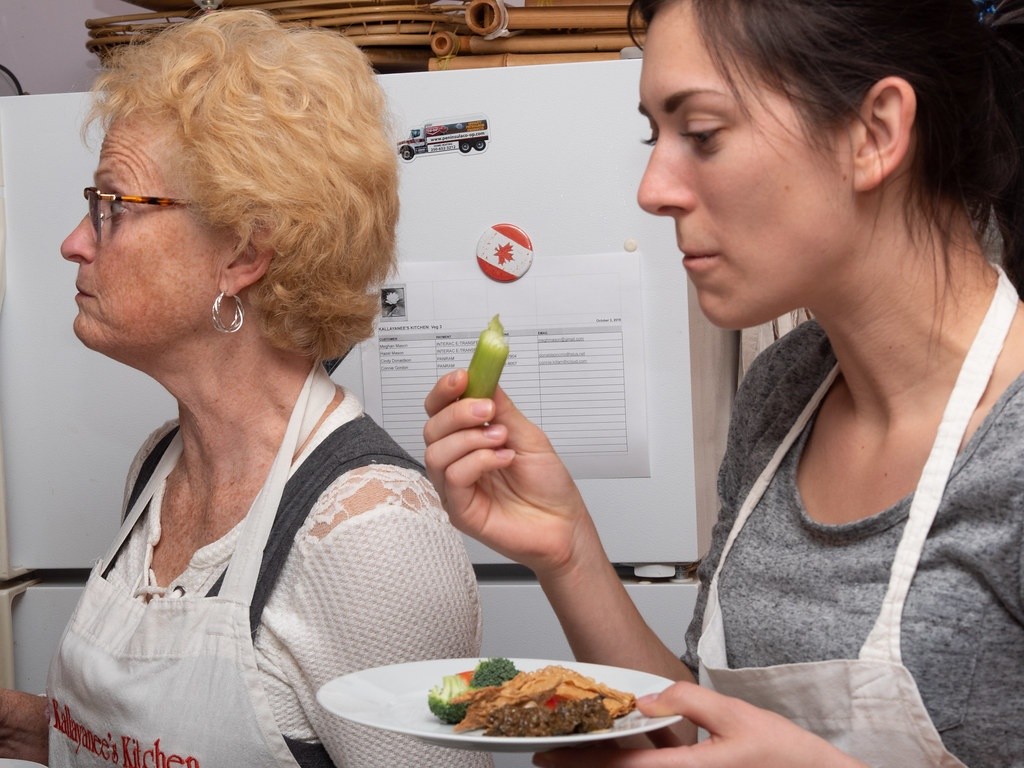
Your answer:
[429,676,473,722]
[470,657,518,688]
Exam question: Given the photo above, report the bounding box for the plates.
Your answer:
[316,656,684,752]
[0,758,48,768]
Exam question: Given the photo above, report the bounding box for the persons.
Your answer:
[1,5,482,767]
[423,0,1024,768]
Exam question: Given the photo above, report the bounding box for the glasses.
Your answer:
[84,187,191,242]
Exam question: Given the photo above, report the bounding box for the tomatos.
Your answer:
[459,671,477,682]
[545,697,566,706]
